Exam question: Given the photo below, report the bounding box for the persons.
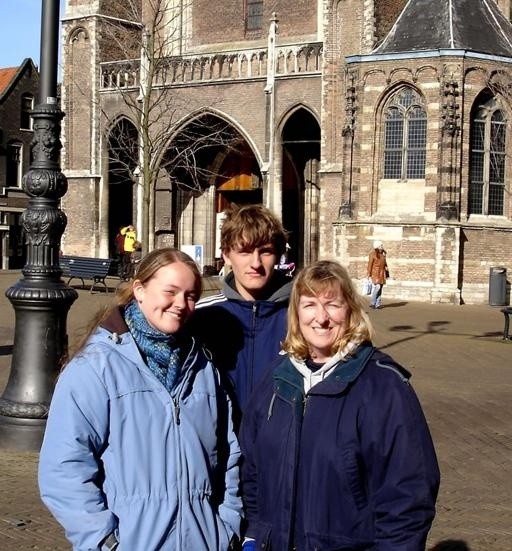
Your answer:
[186,202,296,443]
[36,247,245,550]
[366,239,391,308]
[234,259,441,551]
[273,241,296,278]
[116,224,142,279]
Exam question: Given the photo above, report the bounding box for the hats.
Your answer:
[373,241,383,249]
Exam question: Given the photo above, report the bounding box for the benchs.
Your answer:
[58,252,113,293]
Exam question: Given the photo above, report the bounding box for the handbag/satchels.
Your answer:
[362,280,372,295]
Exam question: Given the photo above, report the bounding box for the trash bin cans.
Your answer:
[489,267,507,306]
